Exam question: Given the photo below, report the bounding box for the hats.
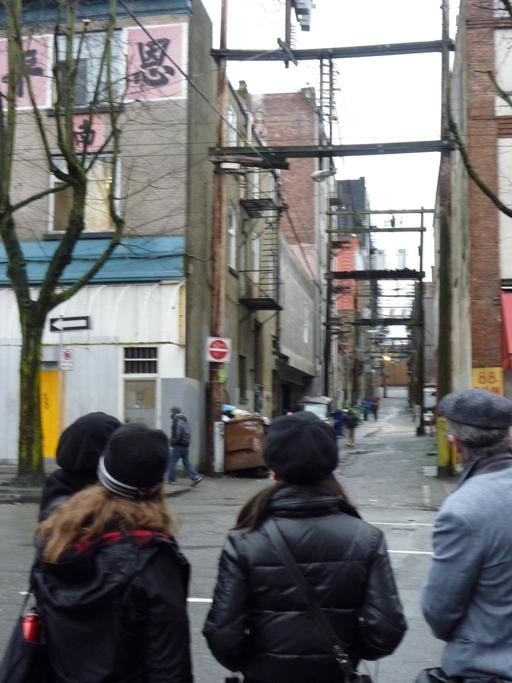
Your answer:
[261,410,338,484]
[439,387,512,429]
[170,405,181,413]
[55,411,171,501]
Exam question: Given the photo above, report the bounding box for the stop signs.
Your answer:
[205,336,233,363]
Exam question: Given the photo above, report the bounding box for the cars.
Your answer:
[285,394,337,428]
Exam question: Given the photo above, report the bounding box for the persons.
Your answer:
[332,394,381,447]
[416,387,512,683]
[203,411,409,683]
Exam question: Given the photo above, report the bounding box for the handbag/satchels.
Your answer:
[0,587,50,682]
[347,670,370,682]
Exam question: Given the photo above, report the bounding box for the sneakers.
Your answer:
[191,475,202,486]
